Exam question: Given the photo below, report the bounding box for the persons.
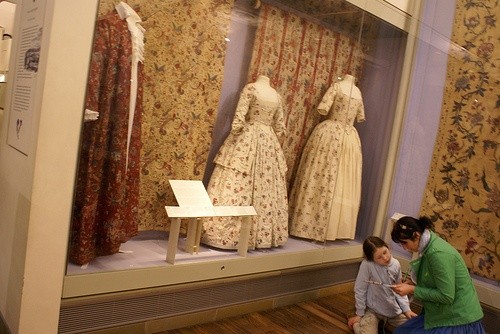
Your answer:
[347,236,419,334]
[288,75,367,243]
[81,1,150,273]
[390,216,487,334]
[199,75,288,251]
